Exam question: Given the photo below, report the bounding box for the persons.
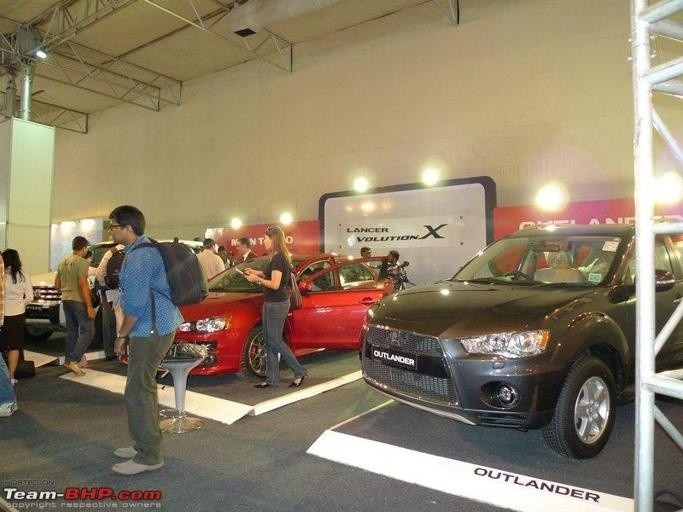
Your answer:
[185,236,256,283]
[242,226,307,389]
[0,247,35,386]
[107,204,185,476]
[1,253,19,418]
[378,249,406,292]
[360,246,373,268]
[52,234,126,376]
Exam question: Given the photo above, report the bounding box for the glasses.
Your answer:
[108,223,127,229]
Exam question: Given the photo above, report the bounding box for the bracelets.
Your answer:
[116,331,127,338]
[256,279,259,285]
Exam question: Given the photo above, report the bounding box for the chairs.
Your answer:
[533,249,585,285]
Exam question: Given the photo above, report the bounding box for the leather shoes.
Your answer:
[254,383,271,388]
[288,371,309,388]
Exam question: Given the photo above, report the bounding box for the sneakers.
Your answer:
[0,400,19,418]
[112,460,165,475]
[63,360,85,376]
[114,446,138,458]
[10,379,18,386]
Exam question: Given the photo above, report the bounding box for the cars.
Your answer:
[165,253,389,383]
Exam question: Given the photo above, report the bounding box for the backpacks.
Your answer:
[129,237,208,306]
[104,246,125,289]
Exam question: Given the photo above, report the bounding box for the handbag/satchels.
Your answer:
[87,276,101,309]
[290,274,302,310]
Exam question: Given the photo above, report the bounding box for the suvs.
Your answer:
[16,240,218,341]
[359,226,682,459]
